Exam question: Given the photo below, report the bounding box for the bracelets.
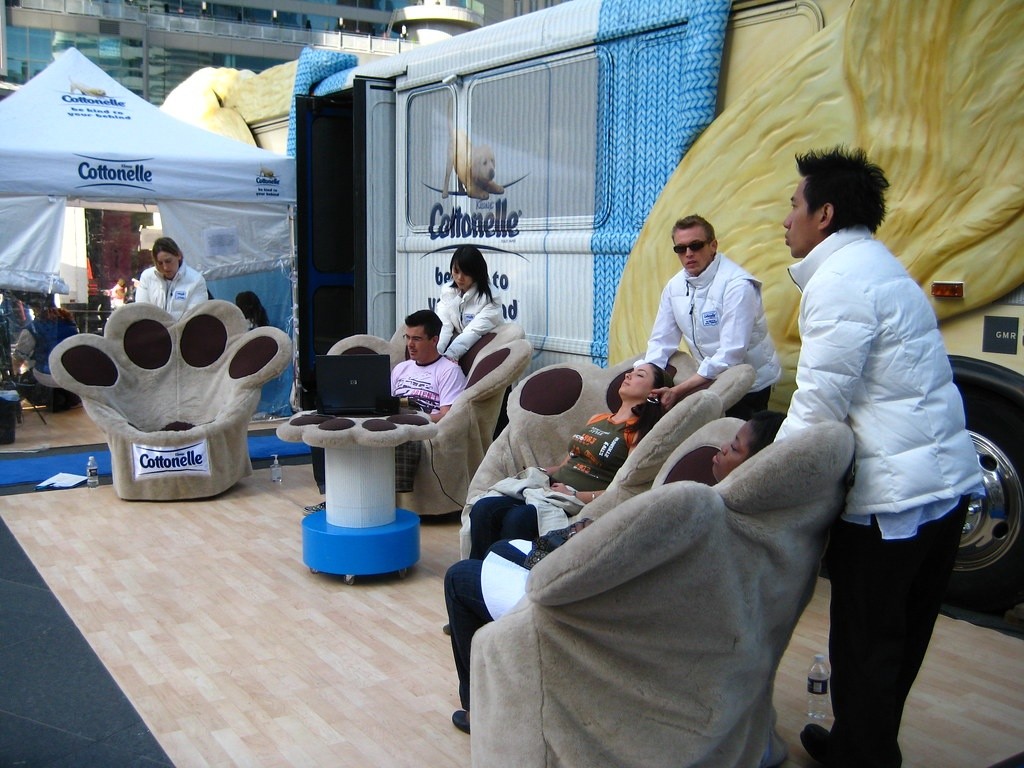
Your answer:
[572,490,576,497]
[591,490,596,500]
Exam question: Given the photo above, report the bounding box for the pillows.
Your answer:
[481,538,533,621]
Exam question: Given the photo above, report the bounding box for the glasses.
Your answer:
[673,238,708,254]
[403,334,432,345]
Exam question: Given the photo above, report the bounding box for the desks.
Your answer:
[274,408,439,586]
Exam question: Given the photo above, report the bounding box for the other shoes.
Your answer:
[46,396,83,409]
[452,710,470,734]
[443,624,451,635]
[800,723,902,768]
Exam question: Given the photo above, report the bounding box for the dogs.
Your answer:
[442,98,505,200]
[68,76,115,100]
[259,162,276,179]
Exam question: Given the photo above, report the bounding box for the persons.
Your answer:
[303,308,466,513]
[773,143,984,768]
[442,362,675,634]
[135,237,209,319]
[433,244,520,439]
[0,287,84,414]
[632,213,781,424]
[445,410,786,738]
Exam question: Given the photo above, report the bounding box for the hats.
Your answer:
[21,290,55,308]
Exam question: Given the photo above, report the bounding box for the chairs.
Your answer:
[469,420,855,768]
[51,299,293,500]
[461,349,756,528]
[327,322,534,517]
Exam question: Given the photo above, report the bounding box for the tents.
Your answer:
[0,47,297,419]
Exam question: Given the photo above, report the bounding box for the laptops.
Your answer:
[315,353,401,416]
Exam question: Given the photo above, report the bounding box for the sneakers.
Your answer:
[303,502,326,516]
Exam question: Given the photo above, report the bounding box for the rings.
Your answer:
[568,531,576,537]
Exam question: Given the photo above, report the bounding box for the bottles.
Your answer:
[87,456,98,488]
[808,653,829,720]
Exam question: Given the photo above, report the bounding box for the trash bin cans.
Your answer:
[0,389,21,445]
